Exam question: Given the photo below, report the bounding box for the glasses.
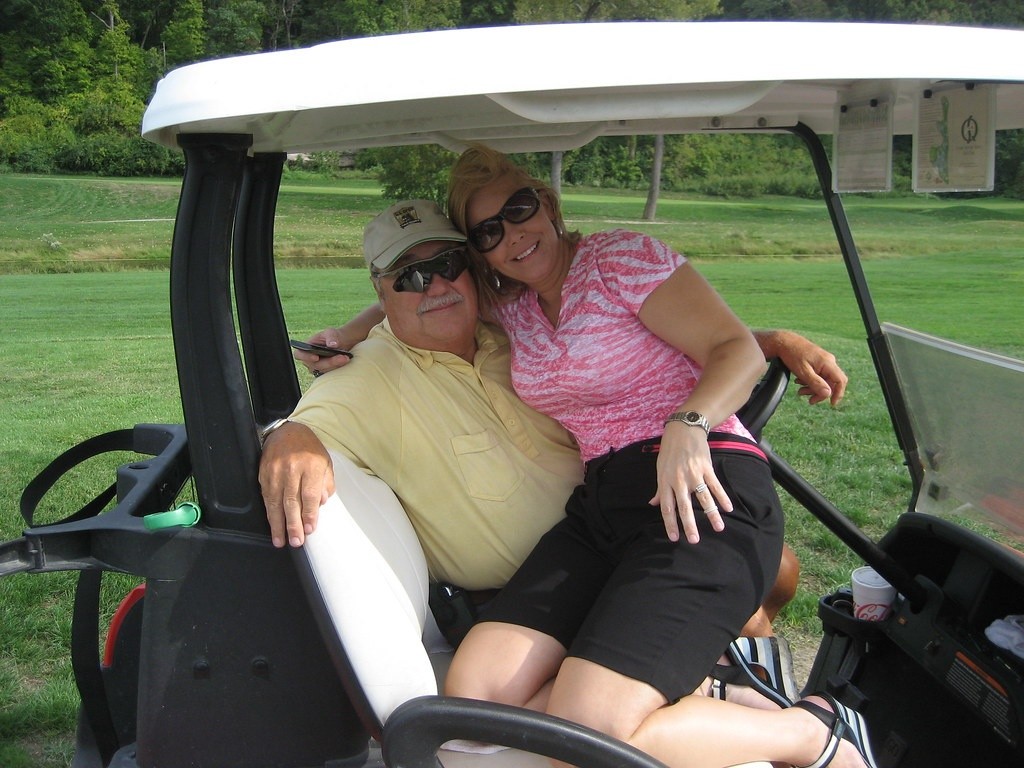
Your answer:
[467,187,540,253]
[374,246,469,293]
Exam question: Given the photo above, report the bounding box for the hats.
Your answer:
[362,199,467,276]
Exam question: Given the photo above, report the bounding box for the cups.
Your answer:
[851,566,897,622]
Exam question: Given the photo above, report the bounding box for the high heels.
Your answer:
[708,637,800,709]
[790,691,877,768]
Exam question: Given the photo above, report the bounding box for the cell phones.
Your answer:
[290,339,353,359]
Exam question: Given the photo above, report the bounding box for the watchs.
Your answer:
[261,418,293,450]
[664,411,711,437]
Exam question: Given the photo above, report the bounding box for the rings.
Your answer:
[694,483,707,494]
[312,368,324,377]
[704,506,718,514]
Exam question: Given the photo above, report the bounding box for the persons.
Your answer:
[258,142,881,767]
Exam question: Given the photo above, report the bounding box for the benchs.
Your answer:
[287,450,775,768]
